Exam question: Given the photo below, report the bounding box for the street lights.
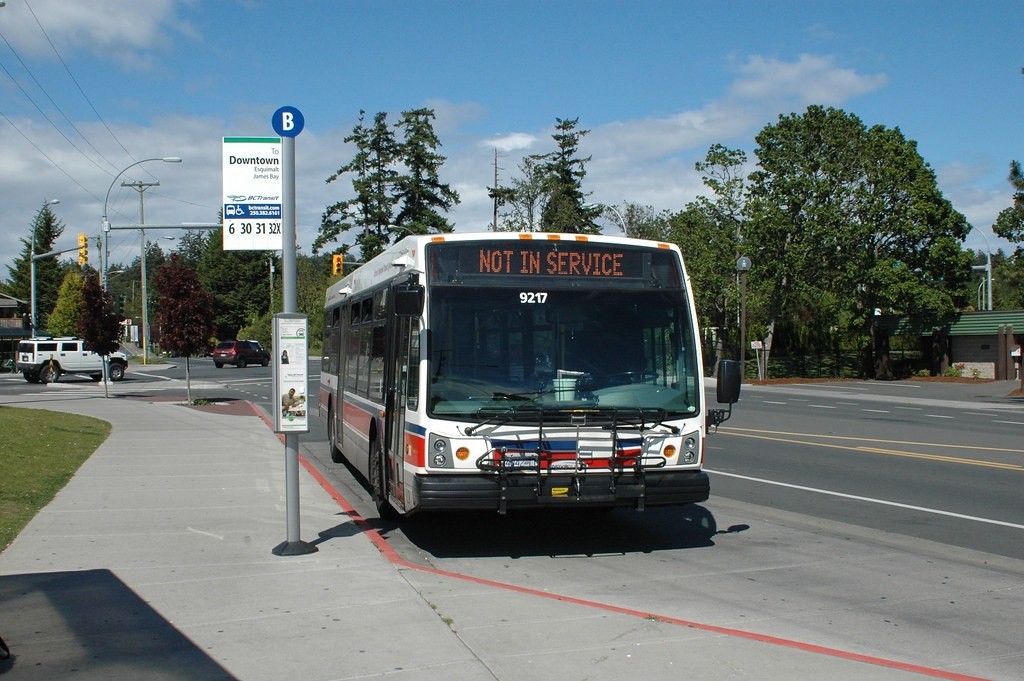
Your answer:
[99,269,125,291]
[101,157,182,318]
[29,199,60,339]
[977,278,996,311]
[140,236,176,365]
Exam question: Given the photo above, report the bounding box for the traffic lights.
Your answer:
[336,257,341,272]
[85,237,88,263]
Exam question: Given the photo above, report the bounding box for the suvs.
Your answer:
[17,337,129,385]
[213,339,271,368]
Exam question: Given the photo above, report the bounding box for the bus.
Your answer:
[318,232,742,523]
